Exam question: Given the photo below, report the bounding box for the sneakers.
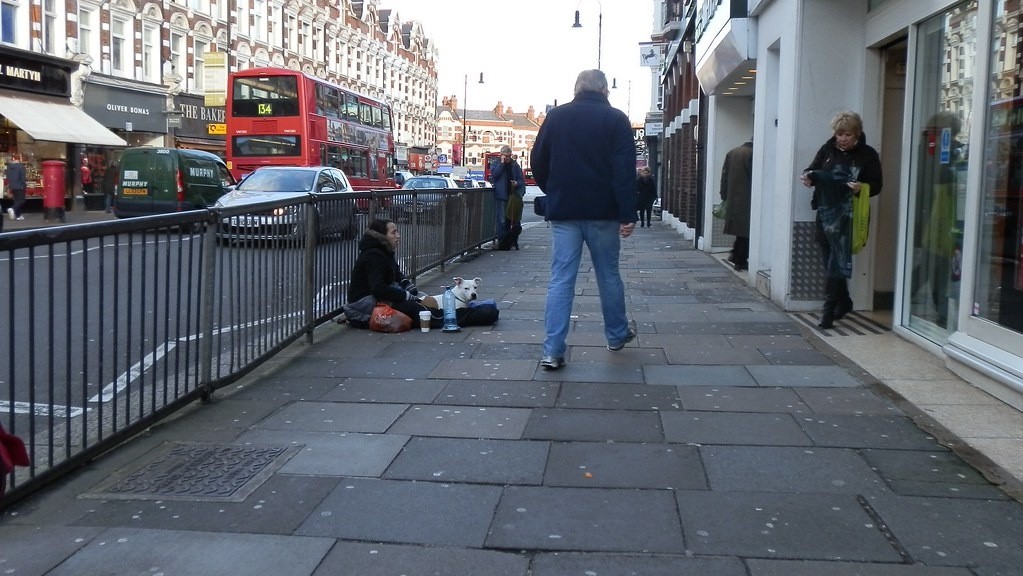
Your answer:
[541,355,566,368]
[607,320,637,351]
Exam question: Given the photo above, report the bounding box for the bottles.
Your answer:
[442,285,457,330]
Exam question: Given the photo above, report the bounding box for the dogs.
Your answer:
[415,277,482,310]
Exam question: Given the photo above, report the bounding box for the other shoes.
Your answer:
[728,254,736,263]
[7,208,14,220]
[16,216,24,221]
[833,297,853,321]
[734,262,748,270]
[818,312,833,328]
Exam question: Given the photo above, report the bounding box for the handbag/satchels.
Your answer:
[506,183,523,223]
[853,183,870,252]
[369,304,412,333]
[921,184,957,258]
[712,200,726,218]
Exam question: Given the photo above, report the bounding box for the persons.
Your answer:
[719,136,754,270]
[6,153,27,221]
[490,145,526,251]
[102,161,118,214]
[636,166,657,227]
[345,218,499,330]
[530,69,639,370]
[911,112,968,320]
[800,110,884,329]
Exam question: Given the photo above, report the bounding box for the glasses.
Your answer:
[606,88,610,96]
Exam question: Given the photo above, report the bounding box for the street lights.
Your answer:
[610,76,633,121]
[570,0,604,69]
[462,66,484,169]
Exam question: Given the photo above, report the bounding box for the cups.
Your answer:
[419,311,431,333]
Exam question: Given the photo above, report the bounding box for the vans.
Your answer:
[110,148,239,234]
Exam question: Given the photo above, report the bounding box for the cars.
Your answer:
[217,166,357,246]
[479,181,493,188]
[394,169,415,184]
[454,180,481,189]
[394,176,461,223]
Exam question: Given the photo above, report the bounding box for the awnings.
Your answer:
[0,88,127,146]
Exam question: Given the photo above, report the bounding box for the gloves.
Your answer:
[400,279,418,296]
[406,291,422,304]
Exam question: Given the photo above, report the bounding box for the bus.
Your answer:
[224,68,400,217]
[482,152,517,181]
[522,168,536,186]
